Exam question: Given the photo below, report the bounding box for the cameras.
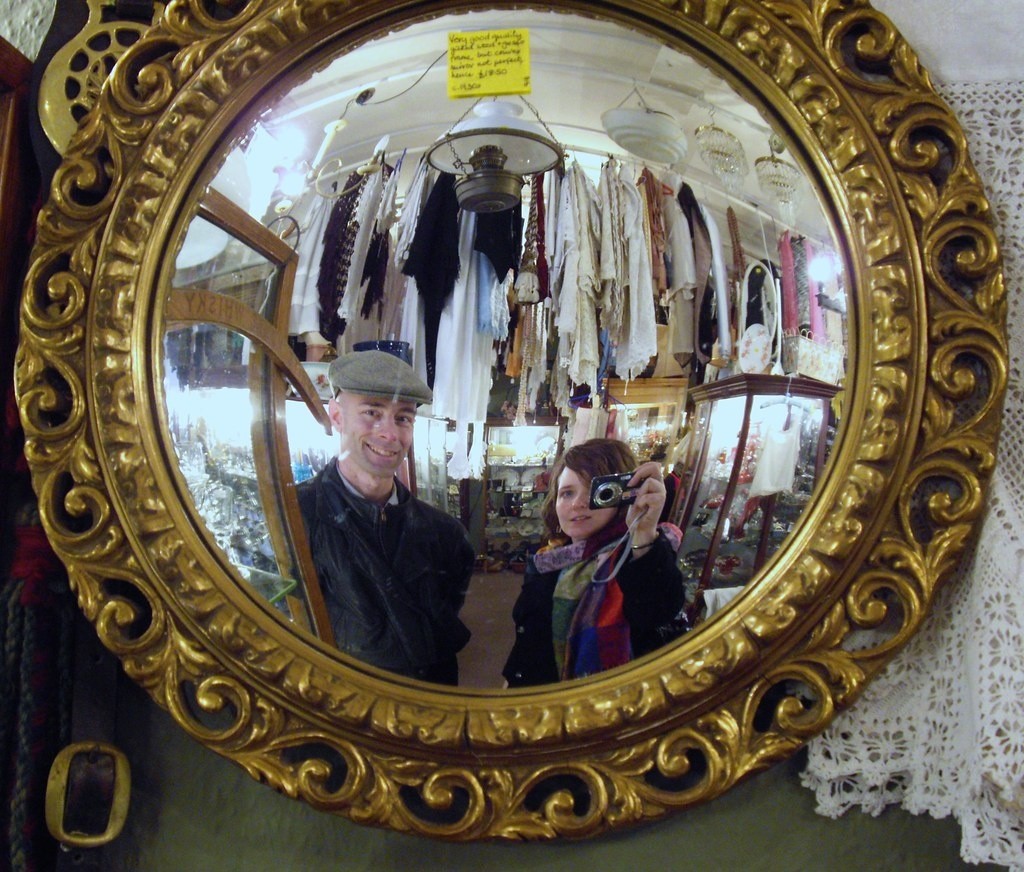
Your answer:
[588,472,644,510]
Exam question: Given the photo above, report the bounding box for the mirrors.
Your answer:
[12,1,1010,840]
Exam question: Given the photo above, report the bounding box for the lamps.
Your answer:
[599,76,687,166]
[753,133,803,206]
[695,101,749,186]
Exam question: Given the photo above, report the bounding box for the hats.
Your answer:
[328,349,433,405]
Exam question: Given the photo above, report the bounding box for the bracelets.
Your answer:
[629,532,660,549]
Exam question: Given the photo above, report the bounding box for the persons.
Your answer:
[293,350,475,689]
[657,462,685,524]
[501,438,685,691]
[650,452,666,462]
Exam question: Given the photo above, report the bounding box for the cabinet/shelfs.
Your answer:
[475,413,569,568]
[605,375,690,473]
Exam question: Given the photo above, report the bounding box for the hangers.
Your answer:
[562,147,675,195]
[392,146,406,176]
